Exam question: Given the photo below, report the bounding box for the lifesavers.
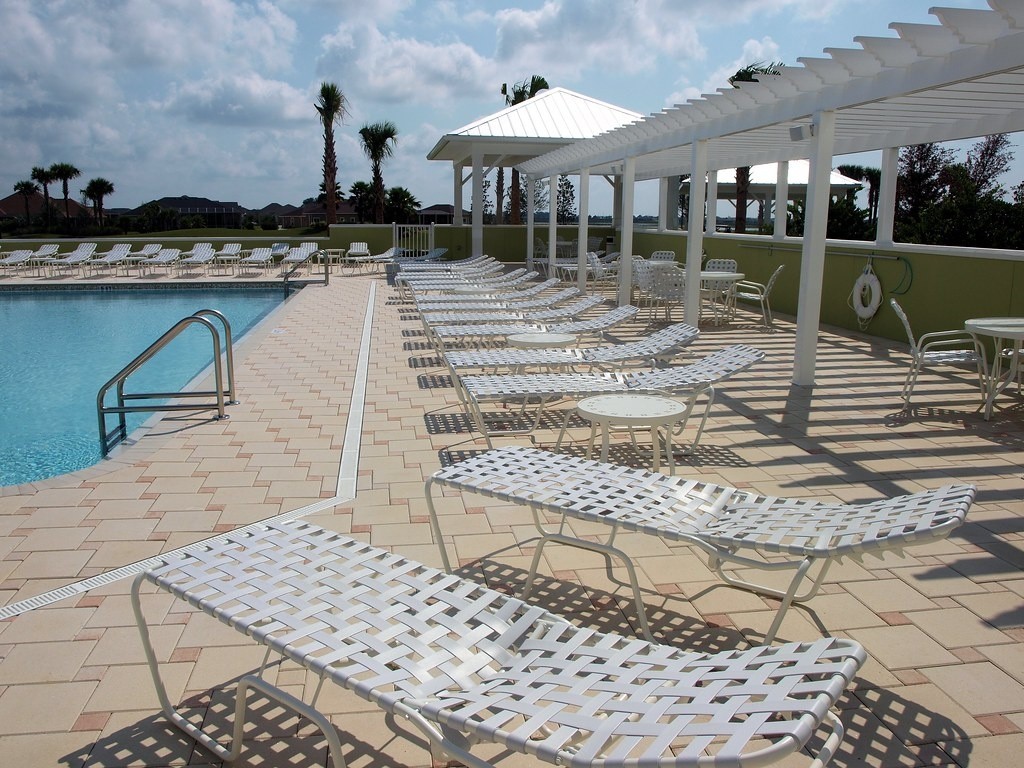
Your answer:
[851,272,884,321]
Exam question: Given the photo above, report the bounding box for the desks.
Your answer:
[577,394,687,476]
[508,333,577,375]
[965,318,1024,421]
[455,287,497,297]
[662,271,745,327]
[634,261,680,306]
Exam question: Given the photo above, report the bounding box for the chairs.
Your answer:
[423,294,603,347]
[443,322,700,421]
[433,304,639,373]
[416,277,561,322]
[526,237,785,330]
[890,296,997,417]
[408,268,527,302]
[412,271,540,306]
[992,337,1024,411]
[460,343,767,451]
[0,242,505,300]
[132,518,867,768]
[424,445,974,666]
[418,286,580,332]
